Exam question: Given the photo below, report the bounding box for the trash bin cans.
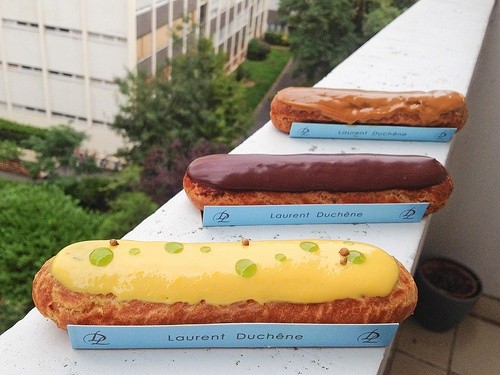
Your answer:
[418,256,484,334]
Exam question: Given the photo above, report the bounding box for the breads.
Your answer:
[183,154,453,219]
[31,238,418,330]
[271,86,466,135]
